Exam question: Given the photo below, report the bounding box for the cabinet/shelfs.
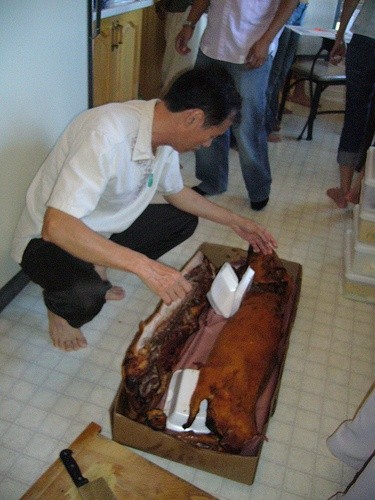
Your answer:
[93,5,207,107]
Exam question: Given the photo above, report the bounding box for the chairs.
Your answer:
[279,39,346,142]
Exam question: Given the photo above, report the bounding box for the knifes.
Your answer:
[60,449,120,500]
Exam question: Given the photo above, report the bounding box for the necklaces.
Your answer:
[148,141,164,189]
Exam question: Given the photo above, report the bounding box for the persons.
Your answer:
[175,0,299,210]
[326,386,375,500]
[11,67,278,352]
[266,0,307,143]
[326,0,375,208]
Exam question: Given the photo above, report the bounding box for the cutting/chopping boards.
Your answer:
[19,422,222,500]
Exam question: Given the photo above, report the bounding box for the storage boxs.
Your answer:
[110,237,304,488]
[343,147,375,304]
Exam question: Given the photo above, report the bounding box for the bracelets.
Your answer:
[183,21,195,29]
